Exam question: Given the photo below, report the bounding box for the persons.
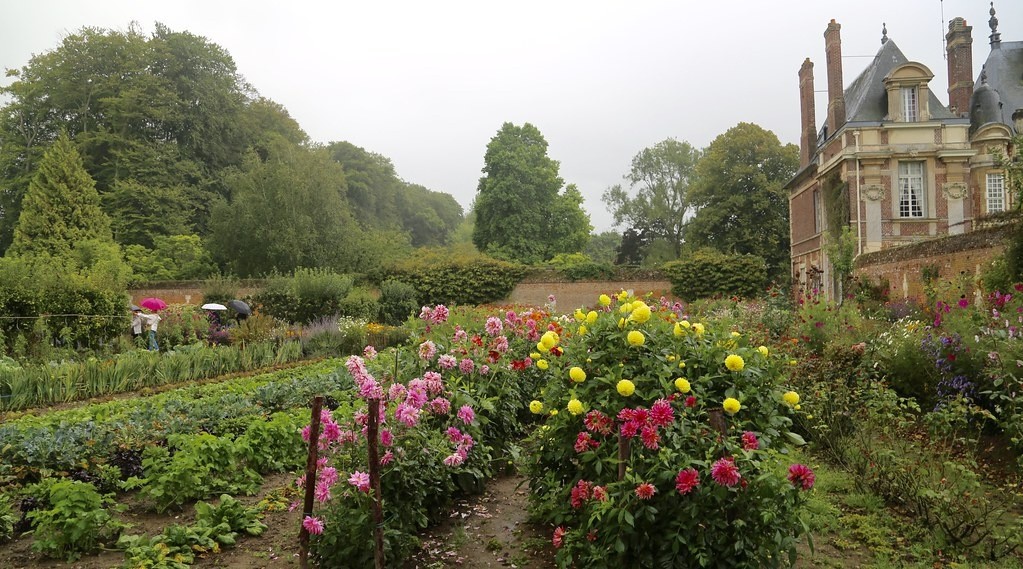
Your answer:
[130,311,142,349]
[134,309,162,352]
[205,310,221,332]
[236,313,247,325]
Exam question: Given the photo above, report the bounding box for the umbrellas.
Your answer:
[230,299,253,315]
[142,297,169,311]
[201,303,227,310]
[130,305,141,311]
